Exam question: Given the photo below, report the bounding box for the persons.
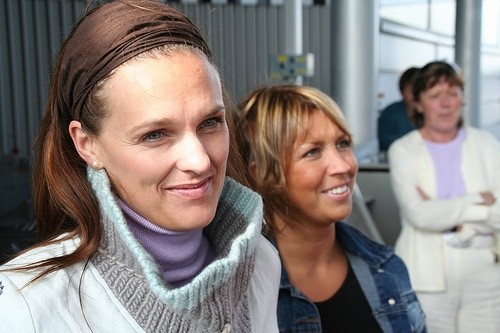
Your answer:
[225,85,430,333]
[375,66,466,154]
[0,0,283,333]
[387,59,500,333]
